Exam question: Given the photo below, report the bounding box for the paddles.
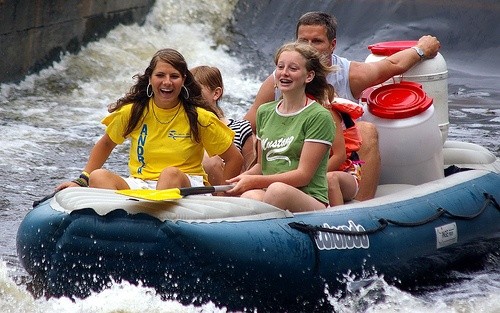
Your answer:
[114,184,234,202]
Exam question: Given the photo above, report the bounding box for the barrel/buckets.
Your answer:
[353,81,445,185]
[360,40,449,147]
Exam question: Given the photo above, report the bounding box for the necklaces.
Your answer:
[152,96,182,124]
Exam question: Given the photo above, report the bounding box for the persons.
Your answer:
[57,48,244,197]
[243,11,440,207]
[189,66,256,197]
[224,43,337,213]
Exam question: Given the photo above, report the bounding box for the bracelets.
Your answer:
[71,170,90,187]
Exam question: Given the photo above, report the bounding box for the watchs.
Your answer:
[411,46,424,63]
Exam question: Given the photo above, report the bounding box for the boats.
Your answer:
[15,141,500,313]
